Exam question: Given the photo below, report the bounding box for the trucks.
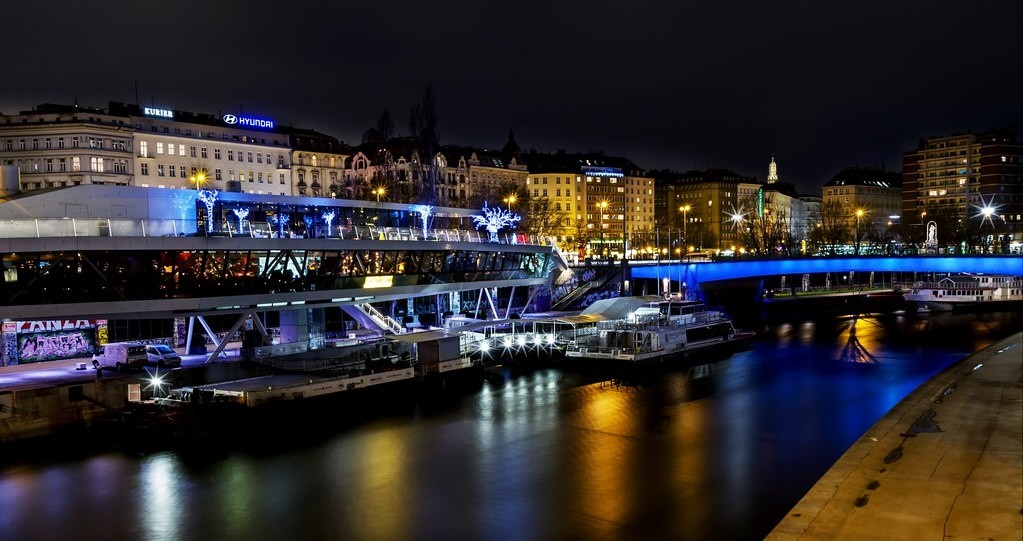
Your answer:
[91,341,149,372]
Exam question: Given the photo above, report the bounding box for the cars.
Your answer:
[146,343,182,369]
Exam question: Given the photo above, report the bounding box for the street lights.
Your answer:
[596,201,608,261]
[855,210,863,256]
[679,205,690,259]
[503,196,515,233]
[980,206,994,257]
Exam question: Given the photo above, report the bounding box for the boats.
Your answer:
[561,229,756,369]
[902,273,1023,312]
